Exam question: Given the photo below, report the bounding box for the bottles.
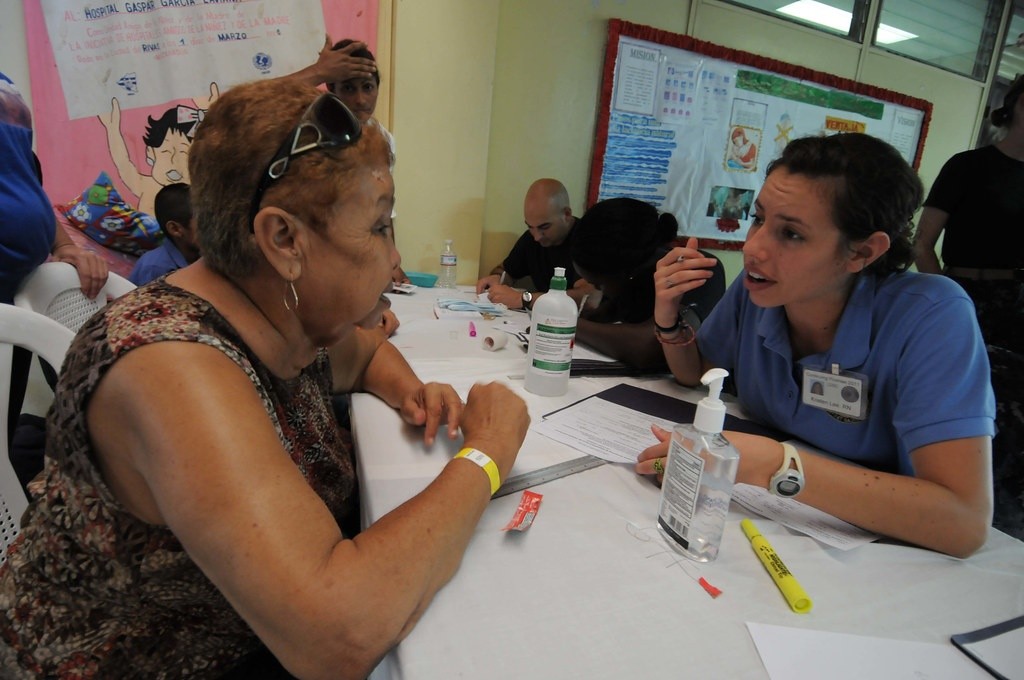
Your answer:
[439,238,462,291]
[523,266,579,397]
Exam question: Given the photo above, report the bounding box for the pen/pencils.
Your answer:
[499,271,506,285]
[741,518,811,614]
[469,322,476,337]
[677,256,684,261]
[433,304,440,320]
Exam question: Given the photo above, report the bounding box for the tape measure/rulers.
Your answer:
[490,454,610,500]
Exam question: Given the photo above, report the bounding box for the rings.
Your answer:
[653,458,664,473]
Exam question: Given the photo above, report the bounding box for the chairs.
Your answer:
[0,262,138,565]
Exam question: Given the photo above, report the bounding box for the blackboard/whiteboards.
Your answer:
[591,18,935,251]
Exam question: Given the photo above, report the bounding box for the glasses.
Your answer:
[247,90,362,236]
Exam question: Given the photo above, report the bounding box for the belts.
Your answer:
[943,264,1024,282]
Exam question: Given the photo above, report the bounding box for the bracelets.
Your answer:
[452,448,501,496]
[653,320,696,347]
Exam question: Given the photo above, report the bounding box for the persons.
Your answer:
[811,382,823,395]
[0,76,531,680]
[127,182,194,287]
[295,32,411,338]
[0,70,111,305]
[911,73,1024,543]
[633,132,997,559]
[707,198,750,220]
[575,197,726,364]
[476,177,594,309]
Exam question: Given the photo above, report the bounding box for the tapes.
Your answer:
[482,332,508,352]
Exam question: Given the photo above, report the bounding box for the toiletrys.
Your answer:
[655,367,741,563]
[522,267,579,398]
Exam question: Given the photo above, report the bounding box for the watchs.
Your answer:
[522,291,533,310]
[768,442,806,499]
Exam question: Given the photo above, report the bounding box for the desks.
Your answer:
[350,282,1024,680]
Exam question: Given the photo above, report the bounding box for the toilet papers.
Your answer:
[481,331,508,351]
